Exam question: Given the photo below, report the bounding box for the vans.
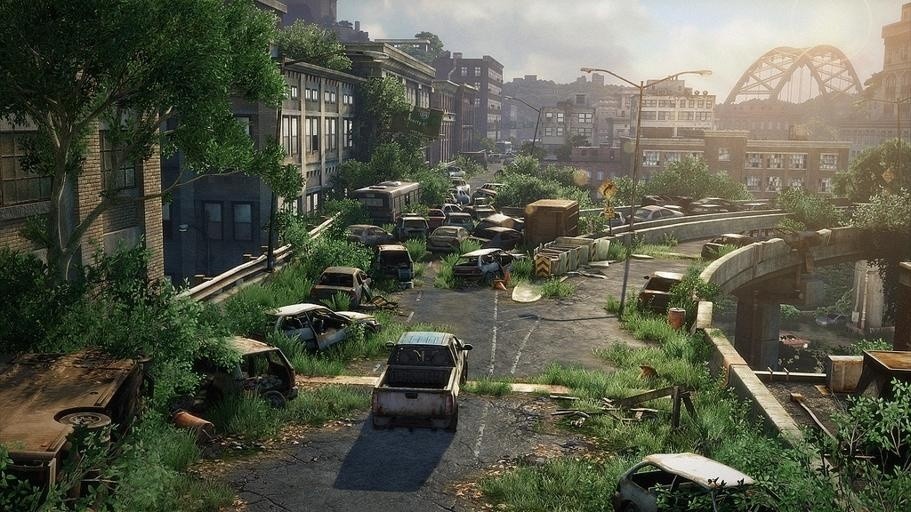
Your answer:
[612,452,756,511]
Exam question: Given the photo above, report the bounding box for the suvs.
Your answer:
[375,245,414,280]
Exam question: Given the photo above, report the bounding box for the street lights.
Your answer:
[852,94,909,186]
[504,95,541,155]
[581,67,711,232]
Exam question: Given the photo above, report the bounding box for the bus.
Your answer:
[1,344,153,502]
[495,141,512,154]
[354,180,424,225]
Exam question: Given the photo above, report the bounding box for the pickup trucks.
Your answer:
[372,332,472,430]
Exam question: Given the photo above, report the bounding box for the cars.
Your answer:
[392,166,524,252]
[346,224,393,245]
[779,335,812,349]
[309,264,372,305]
[699,233,761,259]
[455,246,527,286]
[264,304,381,354]
[598,194,779,223]
[638,271,689,314]
